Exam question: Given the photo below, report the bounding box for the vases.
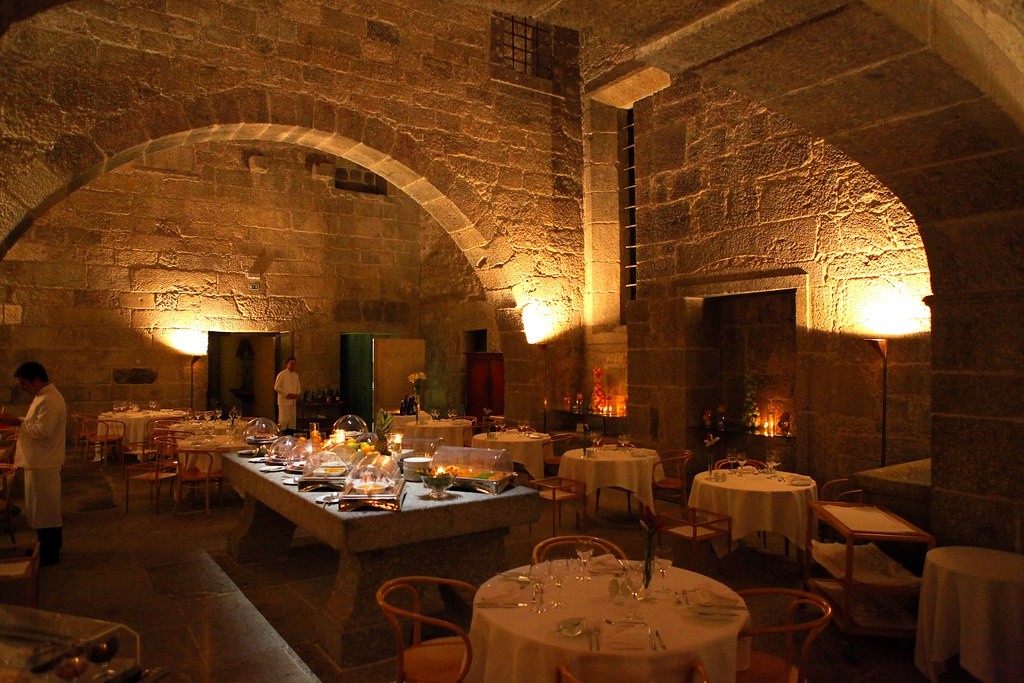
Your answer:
[413,394,420,412]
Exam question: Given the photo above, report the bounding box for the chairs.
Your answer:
[651,448,693,520]
[376,536,832,683]
[455,414,634,451]
[714,459,768,550]
[0,413,224,545]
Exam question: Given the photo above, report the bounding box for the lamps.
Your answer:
[190,355,201,409]
[536,342,548,434]
[865,338,887,469]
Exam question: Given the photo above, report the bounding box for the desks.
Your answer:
[914,545,1024,683]
[541,408,629,435]
[685,422,796,439]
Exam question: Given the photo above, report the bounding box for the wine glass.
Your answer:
[550,558,567,609]
[654,546,673,593]
[530,558,550,613]
[110,401,783,480]
[576,537,592,583]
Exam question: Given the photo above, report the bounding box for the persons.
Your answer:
[273,358,302,435]
[11,361,69,567]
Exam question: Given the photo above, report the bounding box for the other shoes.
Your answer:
[33,551,56,566]
[25,548,39,556]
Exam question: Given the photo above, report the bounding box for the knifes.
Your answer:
[477,605,521,608]
[476,602,527,606]
[655,629,667,650]
[648,625,658,651]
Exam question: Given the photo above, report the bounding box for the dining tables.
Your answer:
[92,409,190,462]
[459,558,752,683]
[176,433,257,504]
[470,433,553,480]
[167,421,255,450]
[222,452,540,671]
[559,446,666,529]
[405,419,473,450]
[687,468,818,570]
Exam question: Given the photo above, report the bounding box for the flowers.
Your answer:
[407,371,427,395]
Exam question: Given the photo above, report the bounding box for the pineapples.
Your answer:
[375,428,387,453]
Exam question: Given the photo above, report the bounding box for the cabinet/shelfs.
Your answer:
[528,476,586,539]
[802,500,935,658]
[658,508,733,570]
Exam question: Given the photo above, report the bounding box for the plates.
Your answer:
[237,450,256,454]
[282,478,298,485]
[248,457,267,462]
[260,466,285,472]
[316,495,339,502]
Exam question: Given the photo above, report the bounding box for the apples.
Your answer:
[742,374,760,428]
[347,438,375,457]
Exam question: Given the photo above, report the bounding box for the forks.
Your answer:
[475,559,750,652]
